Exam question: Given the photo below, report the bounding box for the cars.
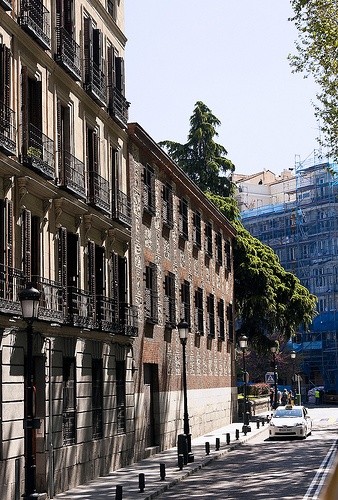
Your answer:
[269,404,314,439]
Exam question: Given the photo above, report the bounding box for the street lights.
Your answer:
[270,340,280,410]
[289,350,298,406]
[15,281,43,500]
[238,333,252,432]
[177,318,194,462]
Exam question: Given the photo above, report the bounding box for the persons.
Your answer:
[315,389,326,405]
[270,387,296,406]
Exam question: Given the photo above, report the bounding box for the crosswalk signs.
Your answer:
[265,372,279,385]
